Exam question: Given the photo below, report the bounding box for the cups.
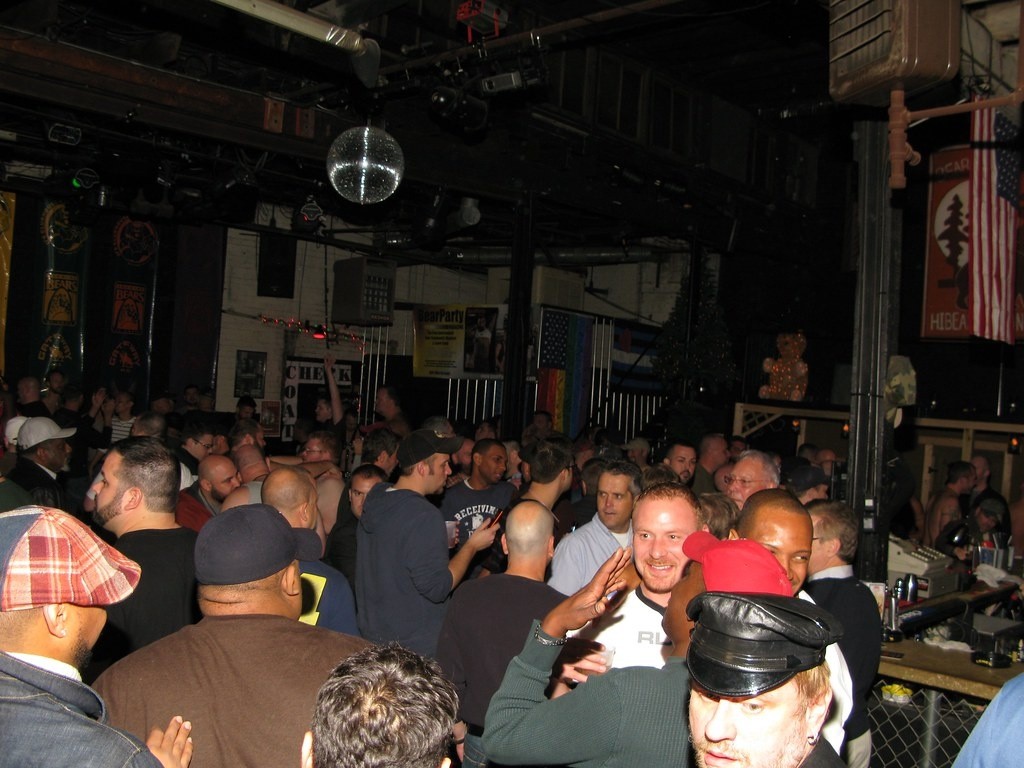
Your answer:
[573,644,615,679]
[445,521,455,548]
[996,546,1013,570]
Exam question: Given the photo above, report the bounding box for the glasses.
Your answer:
[303,447,329,454]
[191,436,214,451]
[724,475,773,488]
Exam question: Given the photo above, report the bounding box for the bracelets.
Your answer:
[451,736,464,744]
[534,624,568,646]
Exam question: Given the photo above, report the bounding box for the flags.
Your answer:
[969,95,1023,344]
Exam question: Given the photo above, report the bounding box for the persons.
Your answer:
[681,590,850,768]
[0,351,1024,768]
[527,325,538,372]
[476,533,795,767]
[0,504,193,768]
[494,332,505,371]
[301,638,462,768]
[466,312,498,372]
[88,501,381,768]
[951,672,1024,767]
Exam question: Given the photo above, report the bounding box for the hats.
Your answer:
[194,503,323,585]
[620,438,650,453]
[396,430,464,468]
[16,416,77,451]
[793,466,829,492]
[5,416,31,444]
[682,531,796,598]
[684,592,845,697]
[0,505,142,612]
[981,499,1003,523]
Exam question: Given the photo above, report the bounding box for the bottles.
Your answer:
[895,574,917,602]
[974,541,996,567]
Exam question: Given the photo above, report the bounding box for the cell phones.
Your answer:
[487,508,504,528]
